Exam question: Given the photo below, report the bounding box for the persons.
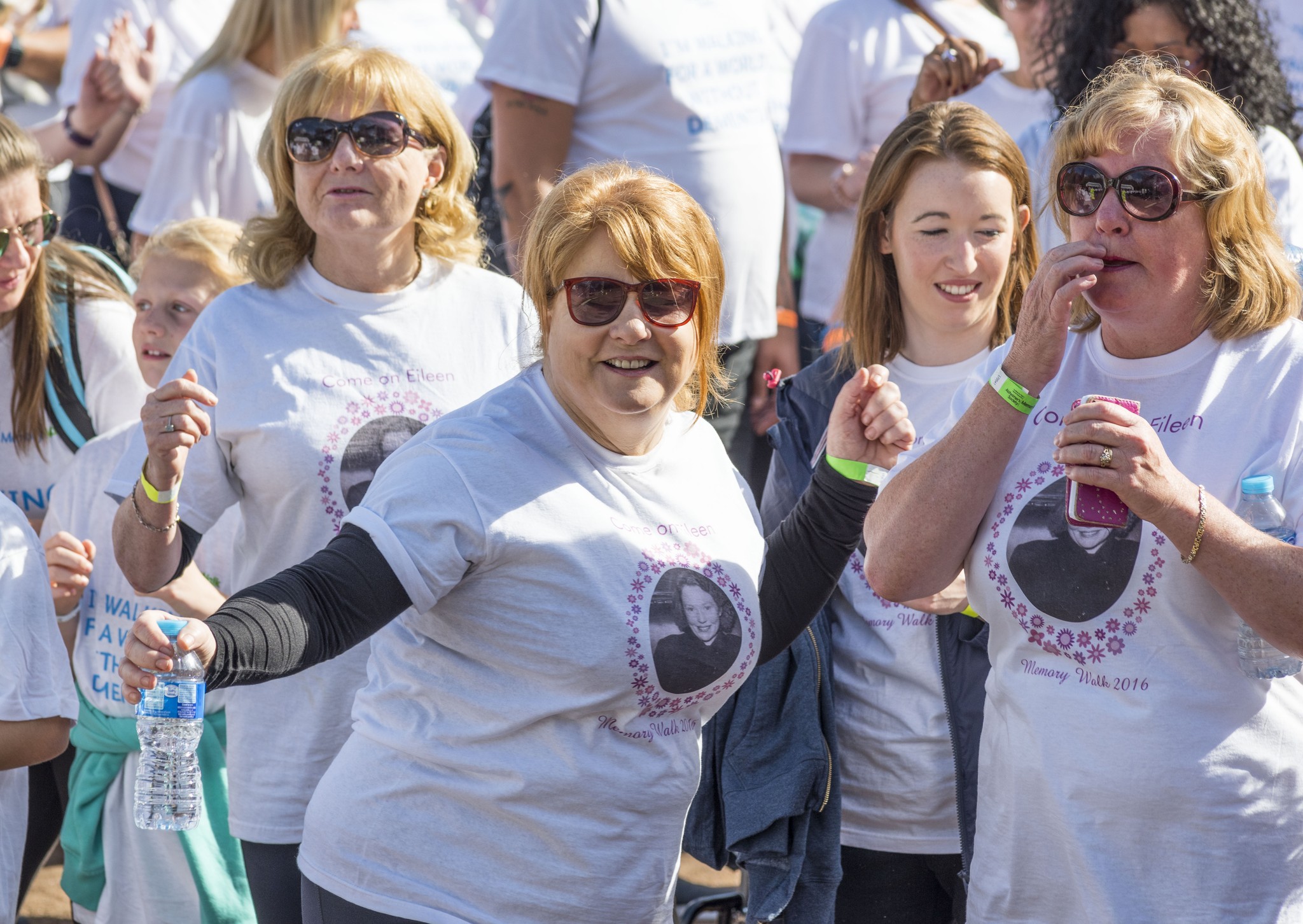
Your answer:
[0,1,1303,924]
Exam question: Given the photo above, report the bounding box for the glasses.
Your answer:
[285,109,437,166]
[552,275,707,329]
[0,201,62,258]
[1055,161,1211,223]
[1111,47,1210,75]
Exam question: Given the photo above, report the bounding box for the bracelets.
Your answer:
[960,603,981,619]
[131,480,179,531]
[824,454,889,487]
[988,365,1042,416]
[1181,483,1206,564]
[63,101,95,148]
[53,601,83,626]
[138,457,185,502]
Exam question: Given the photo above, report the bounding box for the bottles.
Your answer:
[134,619,202,832]
[1234,474,1301,681]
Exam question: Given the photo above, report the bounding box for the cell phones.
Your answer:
[1066,397,1144,528]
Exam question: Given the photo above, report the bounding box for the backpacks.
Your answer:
[37,239,139,456]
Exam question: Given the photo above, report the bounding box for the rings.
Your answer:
[165,415,175,433]
[940,48,958,62]
[1101,446,1113,469]
